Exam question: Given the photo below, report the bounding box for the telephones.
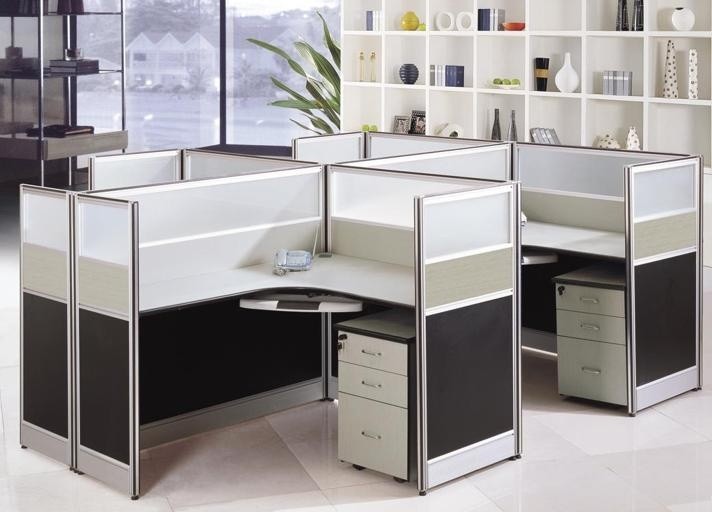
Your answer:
[273,223,320,276]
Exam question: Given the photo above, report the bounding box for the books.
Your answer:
[431,63,464,88]
[479,8,504,31]
[531,127,560,144]
[364,10,380,31]
[26,125,92,138]
[48,66,99,75]
[601,70,632,97]
[49,58,99,66]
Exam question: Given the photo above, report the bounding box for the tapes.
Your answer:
[435,10,455,29]
[456,12,472,30]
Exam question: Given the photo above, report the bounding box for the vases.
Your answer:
[555,52,579,92]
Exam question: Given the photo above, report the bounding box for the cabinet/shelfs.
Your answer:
[552,265,627,406]
[338,0,712,177]
[333,312,416,482]
[0,0,129,189]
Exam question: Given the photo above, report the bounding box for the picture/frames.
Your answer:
[394,115,410,134]
[410,110,426,135]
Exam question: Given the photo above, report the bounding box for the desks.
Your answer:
[291,132,705,417]
[18,151,323,470]
[71,164,524,501]
